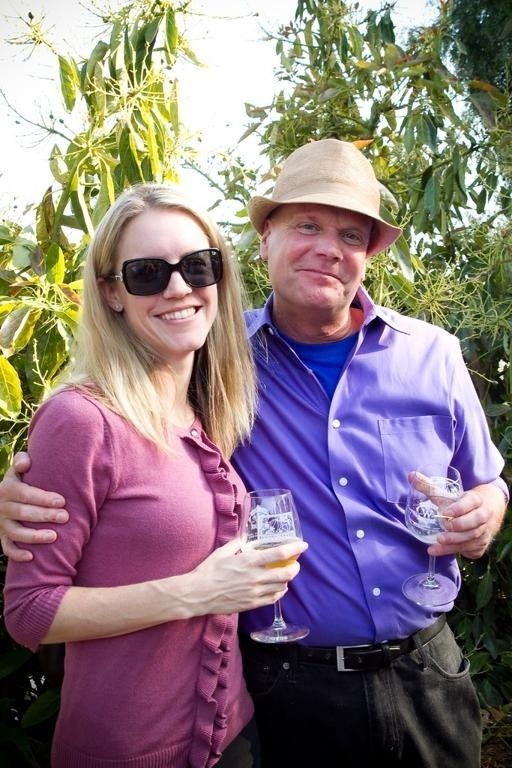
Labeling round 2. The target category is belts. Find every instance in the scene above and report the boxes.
[286,612,447,672]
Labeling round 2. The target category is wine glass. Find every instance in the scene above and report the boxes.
[402,462,464,607]
[239,487,310,643]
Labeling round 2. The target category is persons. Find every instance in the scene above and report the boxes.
[1,181,309,767]
[0,139,510,768]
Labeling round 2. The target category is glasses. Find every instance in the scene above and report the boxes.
[107,247,224,295]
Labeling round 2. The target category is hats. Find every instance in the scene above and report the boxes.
[248,138,402,260]
